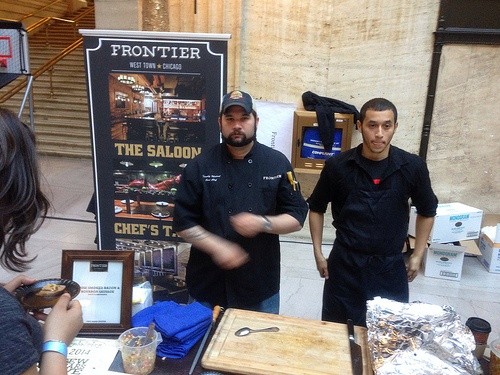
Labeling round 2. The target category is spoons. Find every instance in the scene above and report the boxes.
[235,327,279,337]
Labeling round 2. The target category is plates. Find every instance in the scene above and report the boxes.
[156,201,168,206]
[121,200,134,203]
[16,279,81,309]
[151,211,170,217]
[114,206,122,214]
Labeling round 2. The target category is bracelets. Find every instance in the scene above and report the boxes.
[39,339,68,357]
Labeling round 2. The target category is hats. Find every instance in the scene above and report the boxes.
[222,89,253,114]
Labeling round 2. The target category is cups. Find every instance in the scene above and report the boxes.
[117,327,162,375]
[465,317,491,361]
[489,339,500,375]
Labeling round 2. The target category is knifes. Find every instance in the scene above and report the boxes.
[347,319,363,375]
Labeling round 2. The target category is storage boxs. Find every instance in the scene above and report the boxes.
[409,235,483,283]
[478,226,500,274]
[409,201,484,244]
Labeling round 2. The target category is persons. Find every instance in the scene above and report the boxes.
[305,98,439,328]
[0,108,84,375]
[172,90,309,315]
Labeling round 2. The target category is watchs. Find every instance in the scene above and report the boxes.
[260,214,272,233]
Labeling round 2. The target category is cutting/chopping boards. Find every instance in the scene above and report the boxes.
[201,308,373,375]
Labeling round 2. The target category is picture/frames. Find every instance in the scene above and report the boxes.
[59,250,134,334]
